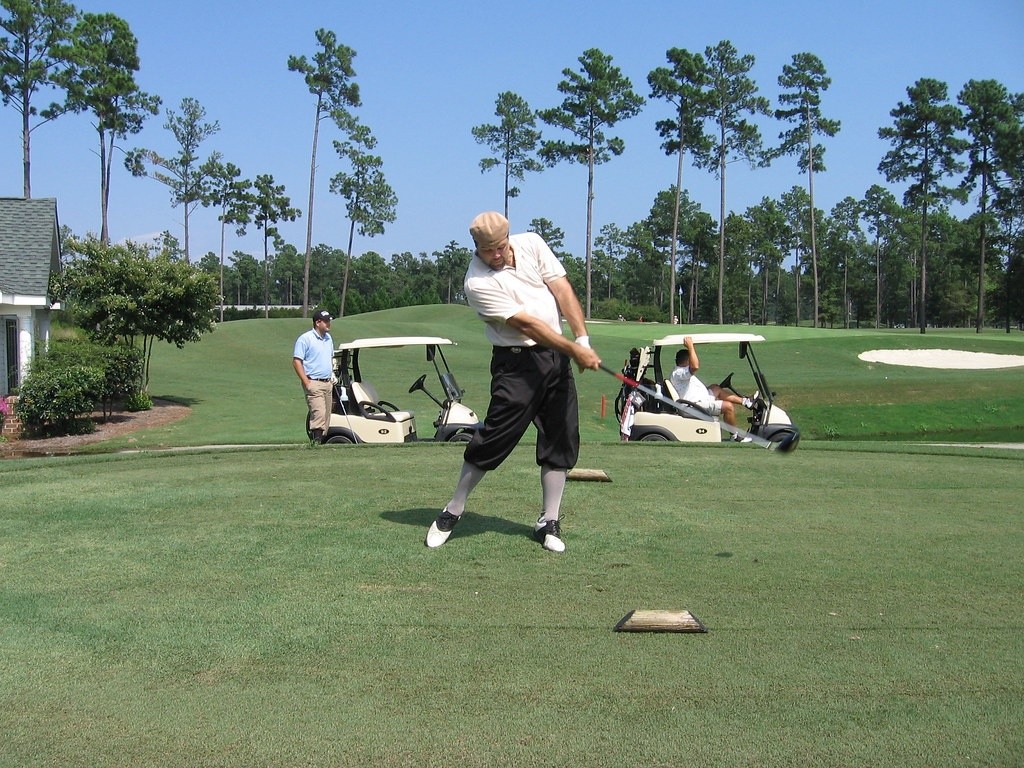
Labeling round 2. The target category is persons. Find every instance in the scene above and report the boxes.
[426,211,602,552]
[670,337,760,442]
[292,310,338,446]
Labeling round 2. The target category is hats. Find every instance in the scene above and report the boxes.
[470,211,509,246]
[313,310,334,322]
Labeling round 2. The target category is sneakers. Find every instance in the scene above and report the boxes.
[533,514,566,552]
[426,501,464,547]
[730,432,752,443]
[745,391,760,410]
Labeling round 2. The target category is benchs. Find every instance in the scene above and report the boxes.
[353,380,415,422]
[664,379,721,421]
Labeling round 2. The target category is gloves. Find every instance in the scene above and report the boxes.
[331,373,338,385]
[308,383,320,396]
[574,336,592,374]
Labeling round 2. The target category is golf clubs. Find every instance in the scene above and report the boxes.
[594,360,799,459]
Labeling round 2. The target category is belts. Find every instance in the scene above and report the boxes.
[308,377,331,382]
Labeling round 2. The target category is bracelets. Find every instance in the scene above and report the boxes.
[575,336,590,349]
